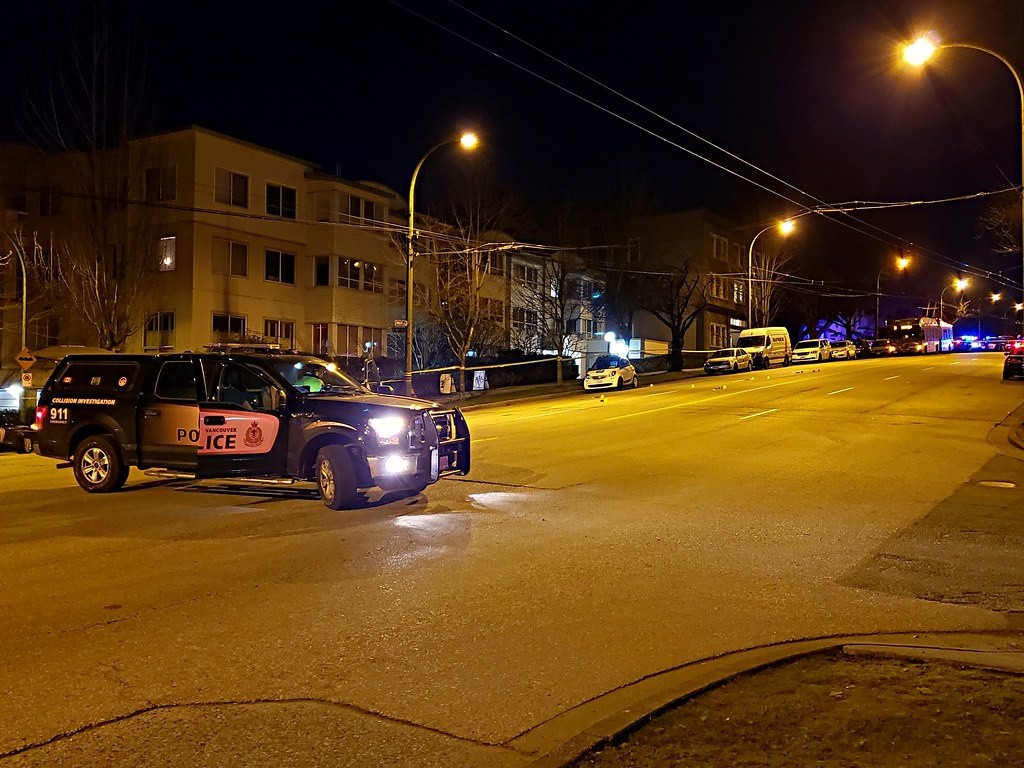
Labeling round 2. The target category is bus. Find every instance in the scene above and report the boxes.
[893,318,954,353]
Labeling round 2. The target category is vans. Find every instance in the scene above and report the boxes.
[736,326,792,369]
[792,339,832,363]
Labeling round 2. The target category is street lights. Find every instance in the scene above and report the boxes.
[394,127,478,396]
[941,281,966,319]
[1001,305,1021,336]
[749,222,793,328]
[604,332,615,353]
[979,295,998,340]
[896,32,1024,298]
[876,258,906,339]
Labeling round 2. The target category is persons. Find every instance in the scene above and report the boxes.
[292,362,327,393]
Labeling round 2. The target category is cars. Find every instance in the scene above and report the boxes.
[1002,353,1024,381]
[583,356,639,391]
[703,347,753,373]
[956,336,1024,351]
[830,341,857,360]
[857,339,922,356]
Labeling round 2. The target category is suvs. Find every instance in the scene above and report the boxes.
[32,352,471,511]
